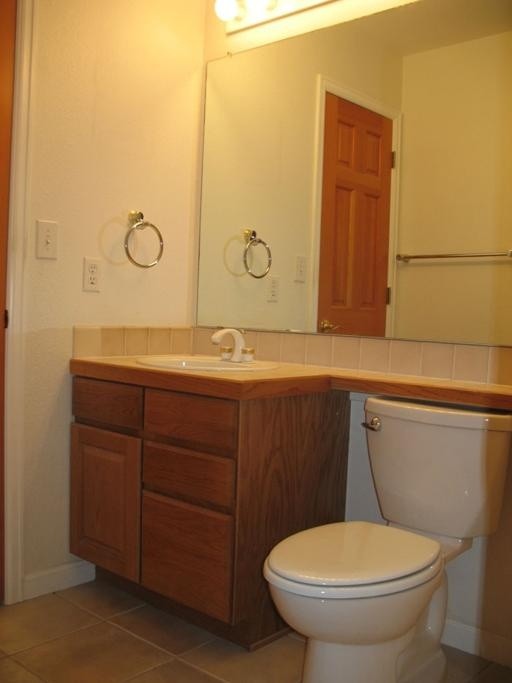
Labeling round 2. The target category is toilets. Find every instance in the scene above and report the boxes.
[262,394,512,683]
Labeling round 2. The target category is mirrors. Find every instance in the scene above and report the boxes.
[195,0,512,348]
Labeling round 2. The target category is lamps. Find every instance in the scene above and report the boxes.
[215,0,332,35]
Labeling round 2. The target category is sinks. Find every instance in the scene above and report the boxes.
[137,359,279,370]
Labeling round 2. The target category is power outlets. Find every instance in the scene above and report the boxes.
[83,256,102,292]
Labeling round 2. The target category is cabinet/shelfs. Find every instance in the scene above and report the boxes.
[70,378,349,653]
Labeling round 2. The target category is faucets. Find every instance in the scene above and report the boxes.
[211,328,246,362]
